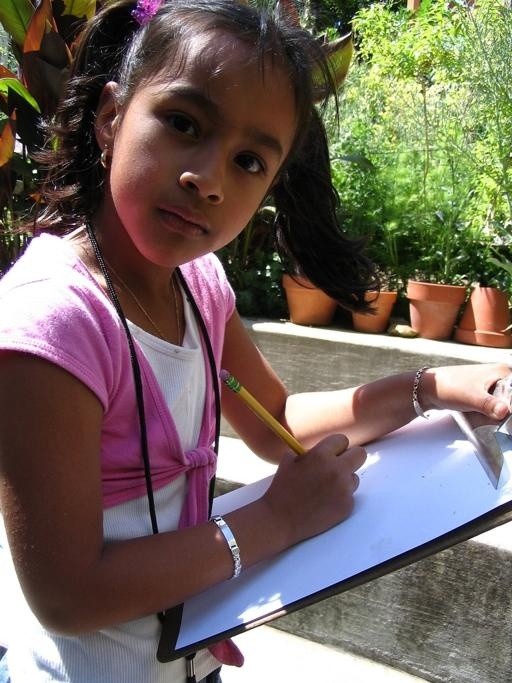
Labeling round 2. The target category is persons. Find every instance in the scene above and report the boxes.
[0,1,511,682]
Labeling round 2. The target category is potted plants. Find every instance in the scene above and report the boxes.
[274,203,511,349]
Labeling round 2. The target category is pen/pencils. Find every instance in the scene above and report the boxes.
[220,369,307,456]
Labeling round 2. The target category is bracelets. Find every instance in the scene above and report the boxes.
[207,514,243,582]
[412,366,432,421]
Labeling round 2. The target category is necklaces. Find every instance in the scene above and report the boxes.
[81,225,180,349]
